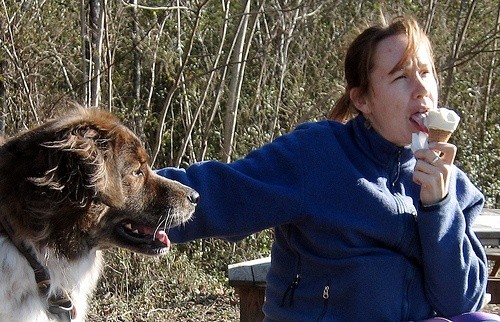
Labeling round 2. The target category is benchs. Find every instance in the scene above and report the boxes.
[226,222,500,322]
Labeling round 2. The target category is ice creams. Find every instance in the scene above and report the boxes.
[424,107,460,156]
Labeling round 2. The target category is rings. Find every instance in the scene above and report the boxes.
[430,156,440,165]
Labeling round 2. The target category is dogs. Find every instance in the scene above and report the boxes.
[0,105,200,322]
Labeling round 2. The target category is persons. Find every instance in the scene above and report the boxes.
[152,17,500,322]
